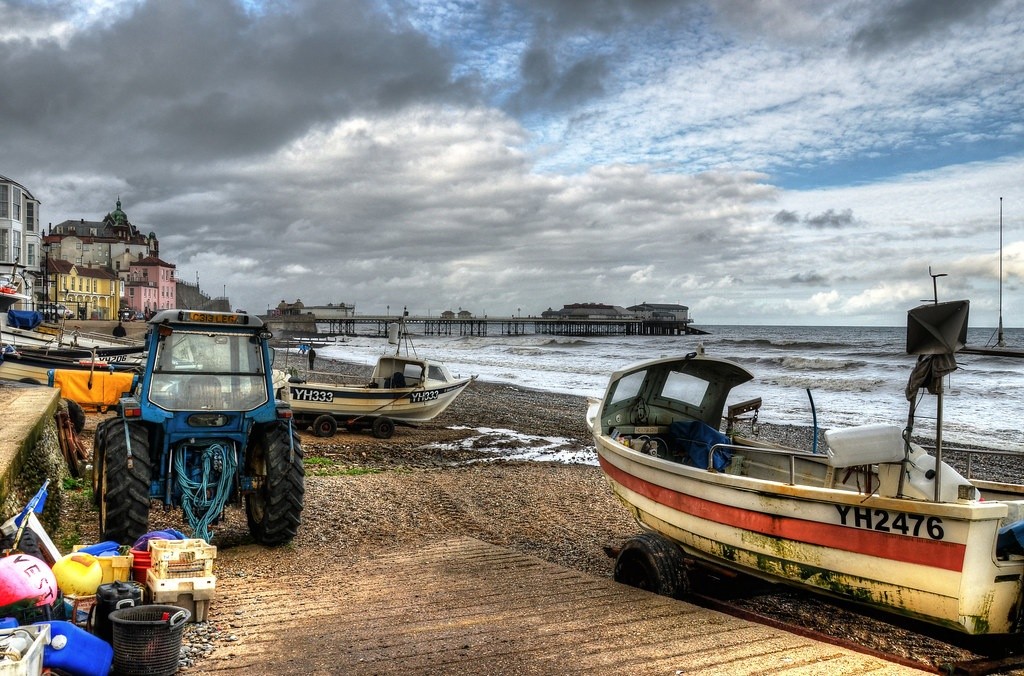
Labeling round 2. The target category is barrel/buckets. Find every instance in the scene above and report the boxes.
[94,580,144,645]
[132,548,151,584]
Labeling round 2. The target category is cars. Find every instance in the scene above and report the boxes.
[36,302,76,320]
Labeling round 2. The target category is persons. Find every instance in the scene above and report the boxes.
[113,322,126,337]
[309,346,316,370]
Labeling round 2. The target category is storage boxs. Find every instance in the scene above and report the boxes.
[73,539,217,631]
[0,592,65,676]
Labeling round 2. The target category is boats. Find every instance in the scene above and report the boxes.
[0,301,480,422]
[582,295,1024,646]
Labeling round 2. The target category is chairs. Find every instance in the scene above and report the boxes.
[185,376,223,410]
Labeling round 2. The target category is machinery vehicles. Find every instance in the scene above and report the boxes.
[90,311,305,547]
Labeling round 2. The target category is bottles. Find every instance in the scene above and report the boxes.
[824,425,906,465]
[32,621,113,676]
[908,456,981,503]
[0,618,18,628]
[878,442,927,500]
